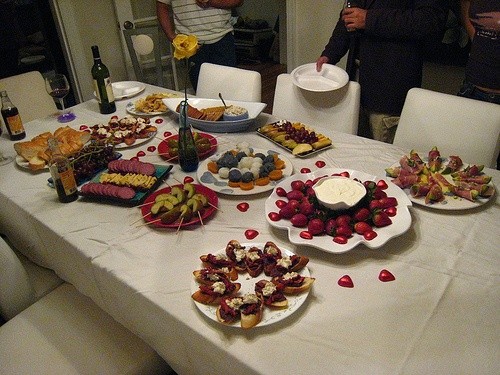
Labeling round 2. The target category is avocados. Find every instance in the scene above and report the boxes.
[168,130,211,155]
[151,183,209,224]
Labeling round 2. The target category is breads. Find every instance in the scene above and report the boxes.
[191,239,315,329]
[91,117,157,145]
[13,124,91,170]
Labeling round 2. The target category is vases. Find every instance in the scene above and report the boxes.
[178,101,199,172]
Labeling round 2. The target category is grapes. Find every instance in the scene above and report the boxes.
[65,144,116,182]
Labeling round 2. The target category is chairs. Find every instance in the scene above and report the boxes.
[196,62,261,103]
[0,237,65,321]
[271,74,360,136]
[393,88,500,169]
[0,281,169,375]
[0,71,57,130]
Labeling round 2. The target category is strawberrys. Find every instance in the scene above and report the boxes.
[279,172,398,235]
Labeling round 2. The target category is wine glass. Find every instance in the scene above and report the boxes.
[45,74,75,123]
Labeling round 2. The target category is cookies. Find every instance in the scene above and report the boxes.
[176,102,216,122]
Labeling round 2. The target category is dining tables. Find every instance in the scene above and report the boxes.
[0,83,500,374]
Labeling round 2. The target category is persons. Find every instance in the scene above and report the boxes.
[455,0,500,105]
[156,0,243,93]
[315,0,450,145]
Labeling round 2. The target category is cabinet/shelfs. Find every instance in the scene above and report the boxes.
[233,28,272,64]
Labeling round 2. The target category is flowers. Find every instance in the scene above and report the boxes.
[172,34,199,163]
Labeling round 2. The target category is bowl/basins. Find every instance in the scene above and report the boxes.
[161,97,267,133]
[312,175,367,210]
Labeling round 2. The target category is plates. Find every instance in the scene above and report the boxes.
[126,95,167,116]
[191,243,311,328]
[105,125,156,148]
[47,151,122,187]
[197,147,293,195]
[255,119,334,158]
[157,131,217,161]
[139,184,220,227]
[265,168,413,255]
[95,80,148,100]
[15,153,51,170]
[76,158,173,205]
[291,62,349,92]
[386,157,496,210]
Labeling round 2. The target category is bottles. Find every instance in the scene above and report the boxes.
[90,45,116,114]
[46,136,81,203]
[0,90,26,141]
